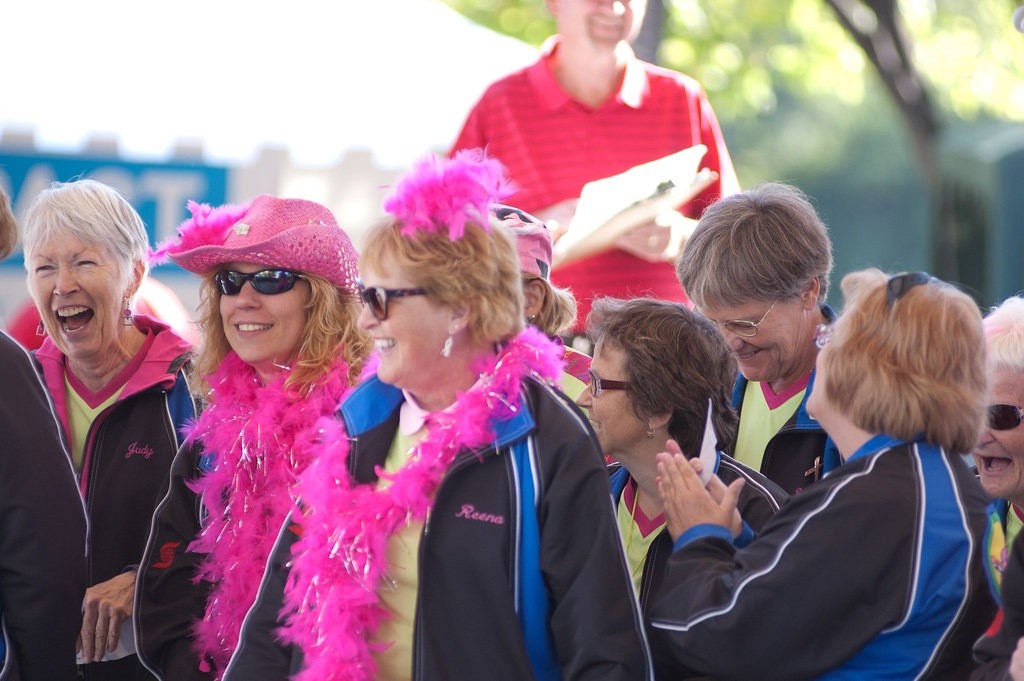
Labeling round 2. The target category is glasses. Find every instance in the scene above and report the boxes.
[987,403,1024,430]
[886,271,930,308]
[214,268,302,295]
[587,369,631,399]
[706,300,779,337]
[816,324,832,348]
[356,284,424,323]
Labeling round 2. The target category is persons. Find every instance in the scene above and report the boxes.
[134,195,377,681]
[489,203,593,404]
[450,0,741,327]
[224,147,650,681]
[972,297,1023,681]
[643,269,1007,681]
[0,329,90,681]
[574,295,789,622]
[20,177,215,681]
[677,182,842,496]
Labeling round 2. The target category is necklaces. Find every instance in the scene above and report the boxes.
[624,486,668,578]
[176,347,355,680]
[270,326,566,681]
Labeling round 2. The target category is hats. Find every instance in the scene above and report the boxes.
[164,195,361,298]
[487,203,555,281]
